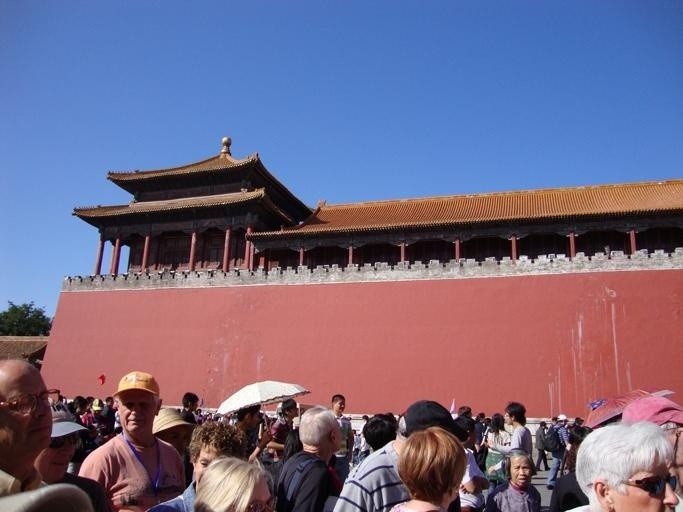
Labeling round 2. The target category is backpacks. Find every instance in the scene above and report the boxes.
[543,426,561,452]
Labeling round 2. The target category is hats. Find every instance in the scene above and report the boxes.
[152,408,191,435]
[47,397,88,438]
[398,400,467,442]
[557,415,570,422]
[112,371,159,398]
[622,395,683,426]
[92,399,104,411]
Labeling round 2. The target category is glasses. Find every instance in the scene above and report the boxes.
[622,476,676,495]
[50,435,81,448]
[249,496,278,512]
[0,389,59,414]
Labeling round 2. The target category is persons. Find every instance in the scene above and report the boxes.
[0,359,488,512]
[456,394,682,512]
